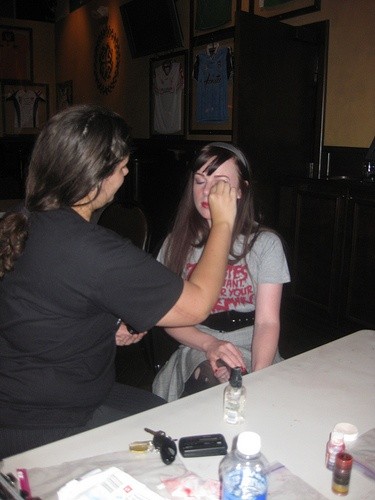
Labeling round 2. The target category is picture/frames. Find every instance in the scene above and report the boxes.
[0,0,322,144]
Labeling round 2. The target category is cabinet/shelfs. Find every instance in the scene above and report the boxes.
[293,170,375,336]
[0,133,39,200]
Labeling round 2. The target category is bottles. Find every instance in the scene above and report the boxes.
[220,431,268,500]
[331,452,353,495]
[223,366,246,425]
[325,431,346,469]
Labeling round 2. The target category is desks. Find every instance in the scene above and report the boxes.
[0,330,375,500]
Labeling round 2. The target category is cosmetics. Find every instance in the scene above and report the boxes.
[224,368,246,423]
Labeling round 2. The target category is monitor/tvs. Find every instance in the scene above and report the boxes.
[119,0,185,61]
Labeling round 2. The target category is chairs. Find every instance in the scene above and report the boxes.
[99,200,159,372]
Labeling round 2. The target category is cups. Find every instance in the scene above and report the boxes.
[331,423,359,446]
[360,159,375,184]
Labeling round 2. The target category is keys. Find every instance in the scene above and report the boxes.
[143,426,177,465]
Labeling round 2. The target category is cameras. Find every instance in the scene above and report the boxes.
[178,433,229,459]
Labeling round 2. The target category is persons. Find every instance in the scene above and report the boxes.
[152,143,291,399]
[0,104,237,455]
